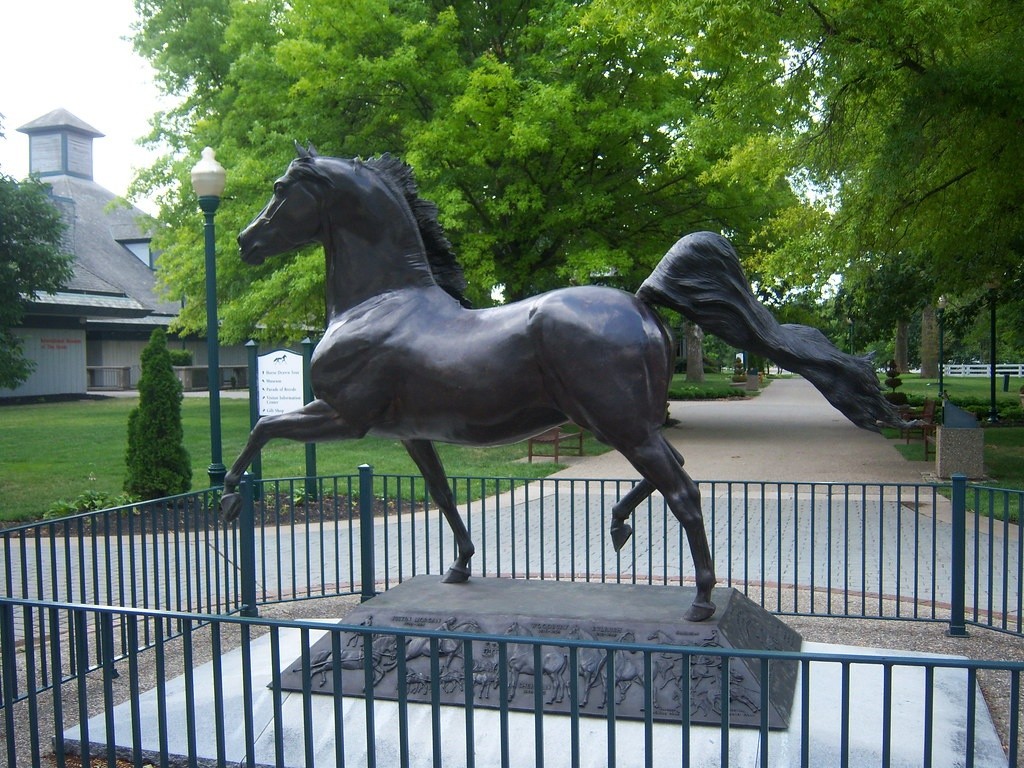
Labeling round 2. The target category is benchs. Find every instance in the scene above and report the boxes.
[922,423,936,461]
[898,397,936,446]
[527,425,586,464]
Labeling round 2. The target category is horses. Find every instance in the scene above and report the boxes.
[310,618,762,717]
[216,140,930,622]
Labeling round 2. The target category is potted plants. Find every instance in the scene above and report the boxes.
[729,357,747,391]
[1019,384,1024,404]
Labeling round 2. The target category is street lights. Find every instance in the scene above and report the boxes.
[189,145,228,510]
[847,317,854,354]
[937,296,947,398]
[983,271,1001,422]
